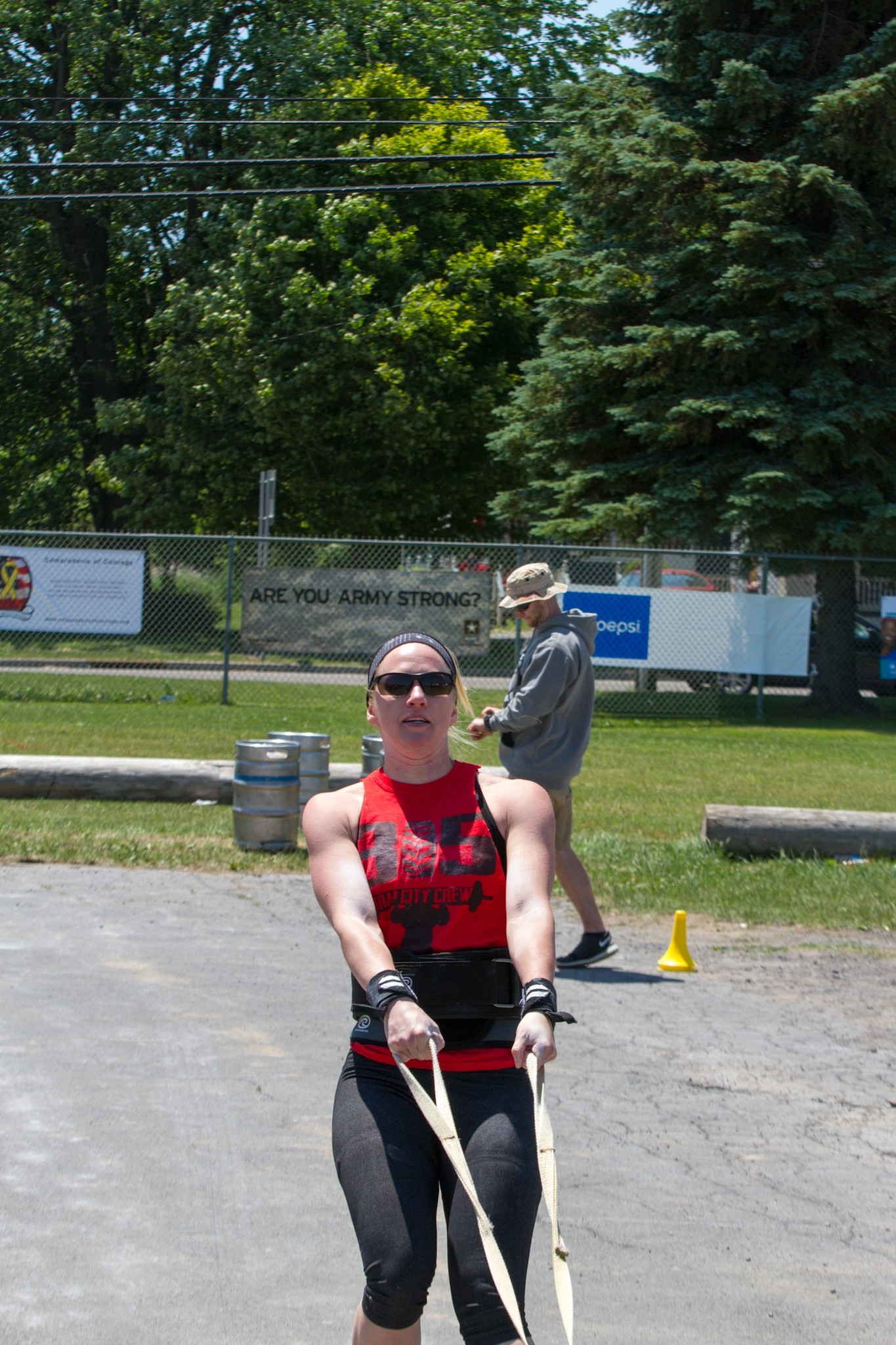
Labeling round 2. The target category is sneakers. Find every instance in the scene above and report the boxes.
[556,930,619,967]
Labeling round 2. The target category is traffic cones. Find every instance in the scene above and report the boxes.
[657,909,698,974]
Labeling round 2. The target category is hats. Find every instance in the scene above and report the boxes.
[498,561,569,608]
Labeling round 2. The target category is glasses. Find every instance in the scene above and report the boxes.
[513,601,532,613]
[367,671,456,698]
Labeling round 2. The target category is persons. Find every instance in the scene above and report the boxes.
[451,550,507,626]
[150,557,204,577]
[303,632,557,1345]
[879,612,896,679]
[467,563,619,966]
[735,555,778,594]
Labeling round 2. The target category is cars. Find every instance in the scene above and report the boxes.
[685,596,882,695]
[617,569,715,591]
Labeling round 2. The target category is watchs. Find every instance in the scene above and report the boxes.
[484,714,492,731]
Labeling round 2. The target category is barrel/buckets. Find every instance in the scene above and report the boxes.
[231,740,301,852]
[359,733,386,780]
[267,732,331,807]
